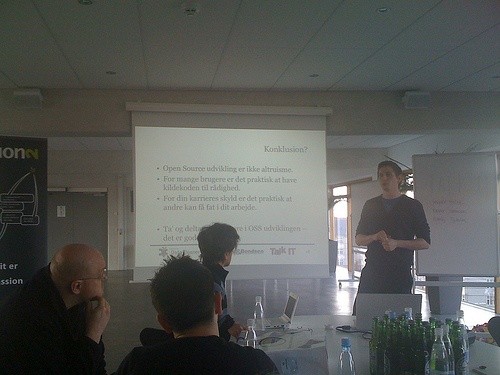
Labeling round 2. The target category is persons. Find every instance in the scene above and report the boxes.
[352,160,432,317]
[0,242,109,375]
[114,222,280,375]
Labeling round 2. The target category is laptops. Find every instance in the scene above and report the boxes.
[262,292,299,328]
[355,293,422,331]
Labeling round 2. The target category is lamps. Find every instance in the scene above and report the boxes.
[401,90,436,110]
[11,87,43,109]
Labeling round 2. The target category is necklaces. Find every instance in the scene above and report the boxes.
[175,332,193,339]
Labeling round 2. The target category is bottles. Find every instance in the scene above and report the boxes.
[245,318,256,347]
[369,306,470,375]
[337,338,355,374]
[253,295,263,329]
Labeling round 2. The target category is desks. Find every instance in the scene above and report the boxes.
[228,315,500,375]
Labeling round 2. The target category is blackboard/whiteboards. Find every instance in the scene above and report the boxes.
[350,179,383,248]
[411,151,500,277]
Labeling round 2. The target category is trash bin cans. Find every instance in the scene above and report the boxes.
[426,277,464,317]
[329,240,338,275]
